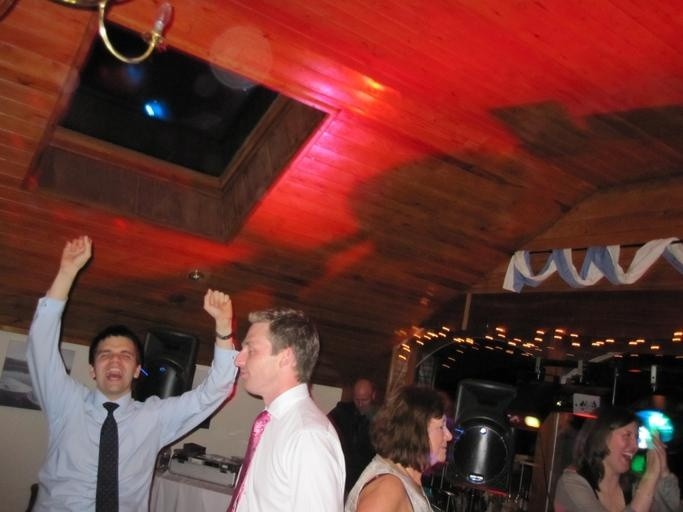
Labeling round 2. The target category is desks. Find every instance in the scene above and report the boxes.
[149,467,234,512]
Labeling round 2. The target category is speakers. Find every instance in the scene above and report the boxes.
[131,329,199,403]
[447,377,523,495]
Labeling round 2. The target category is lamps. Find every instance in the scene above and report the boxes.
[497,379,567,512]
[57,0,174,66]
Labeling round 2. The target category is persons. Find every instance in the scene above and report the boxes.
[223,306,348,511]
[551,404,681,512]
[342,383,454,512]
[324,375,383,506]
[21,234,240,511]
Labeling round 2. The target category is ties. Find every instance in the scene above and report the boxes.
[95,401,119,512]
[225,409,272,512]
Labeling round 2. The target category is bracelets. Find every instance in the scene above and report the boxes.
[213,330,233,340]
